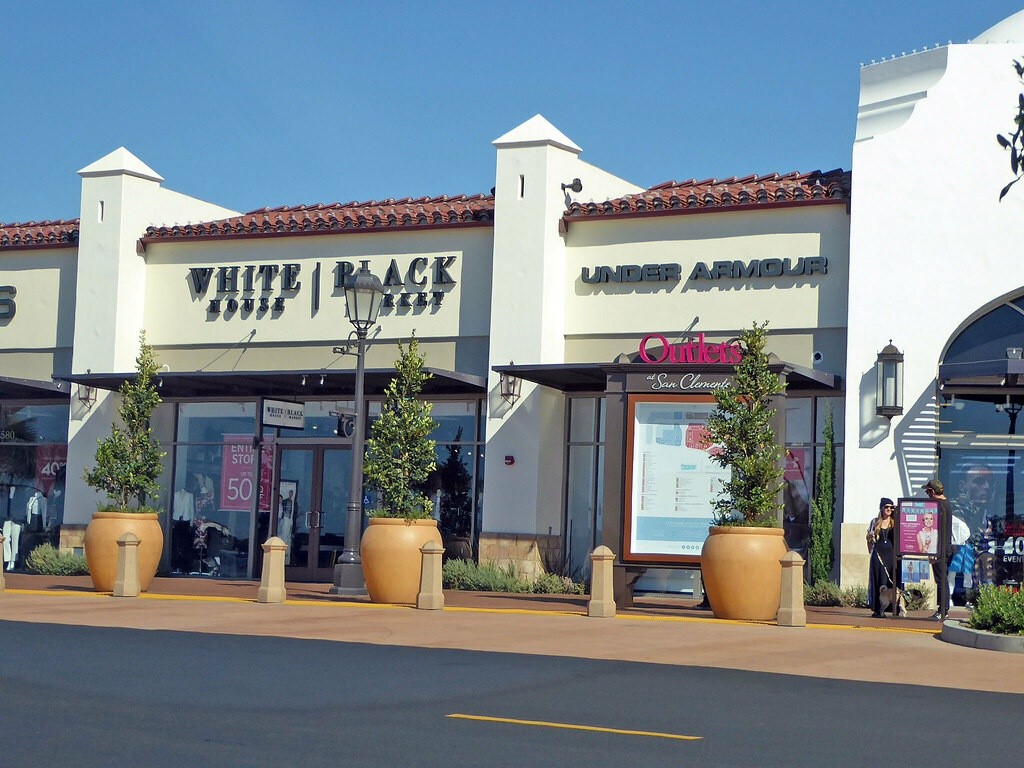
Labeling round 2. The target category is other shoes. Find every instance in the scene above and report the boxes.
[933,611,949,619]
[872,611,886,618]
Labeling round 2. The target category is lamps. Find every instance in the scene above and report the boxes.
[875,339,904,419]
[501,359,524,408]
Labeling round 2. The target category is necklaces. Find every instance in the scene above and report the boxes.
[881,523,889,543]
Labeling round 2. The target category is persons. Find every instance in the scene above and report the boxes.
[948,460,997,593]
[867,498,904,618]
[26,491,47,572]
[193,487,213,576]
[947,517,976,606]
[172,488,194,574]
[282,490,293,559]
[277,495,283,537]
[917,512,938,553]
[45,489,63,548]
[1,486,25,571]
[921,479,954,620]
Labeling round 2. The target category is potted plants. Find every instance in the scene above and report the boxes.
[359,329,443,605]
[85,330,169,594]
[698,319,794,623]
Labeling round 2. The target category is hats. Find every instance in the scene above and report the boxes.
[921,479,943,494]
[879,497,893,508]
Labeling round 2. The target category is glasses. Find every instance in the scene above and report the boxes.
[885,506,894,509]
[925,490,931,494]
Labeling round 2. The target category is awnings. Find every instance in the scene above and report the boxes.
[0,376,69,398]
[53,368,487,395]
[492,342,842,392]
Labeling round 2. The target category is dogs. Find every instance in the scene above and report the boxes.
[879,585,922,618]
